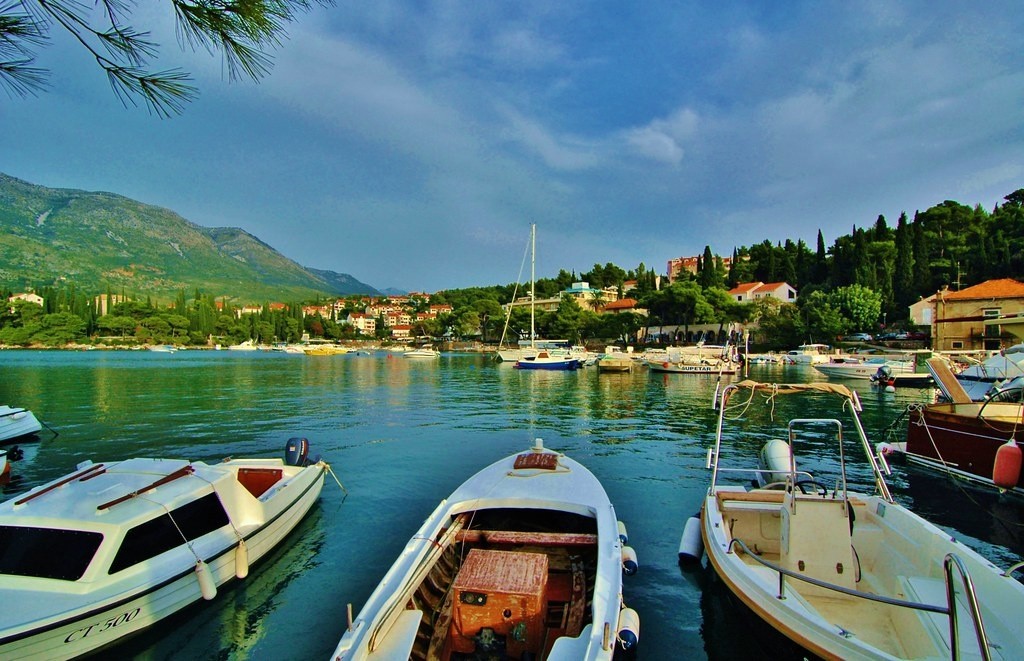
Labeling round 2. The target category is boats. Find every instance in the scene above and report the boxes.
[0,438,345,661]
[678,380,1024,661]
[0,405,42,486]
[403,344,440,358]
[228,341,348,356]
[329,438,640,661]
[146,345,177,352]
[491,224,1024,557]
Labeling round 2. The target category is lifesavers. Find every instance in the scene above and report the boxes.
[723,358,730,363]
[677,363,684,369]
